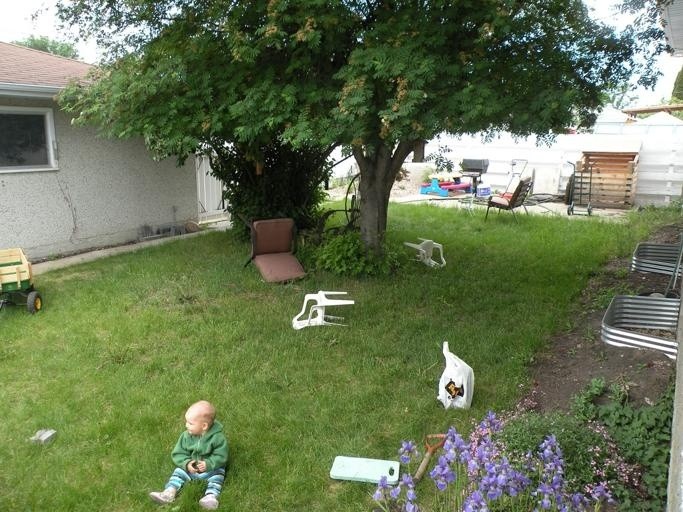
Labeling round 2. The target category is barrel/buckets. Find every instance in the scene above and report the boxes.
[477,184,492,197]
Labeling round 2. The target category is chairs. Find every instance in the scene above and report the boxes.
[292,289,357,332]
[401,234,447,271]
[484,175,534,225]
[233,208,307,285]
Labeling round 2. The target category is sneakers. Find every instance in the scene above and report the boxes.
[198,493,220,510]
[149,491,175,506]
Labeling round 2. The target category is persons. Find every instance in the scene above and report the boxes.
[148,399,231,510]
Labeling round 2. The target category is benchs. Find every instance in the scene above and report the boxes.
[440,183,473,197]
[419,181,451,194]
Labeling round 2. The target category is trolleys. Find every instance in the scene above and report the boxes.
[565,159,599,216]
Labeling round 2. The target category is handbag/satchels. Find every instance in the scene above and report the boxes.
[437,341,475,410]
